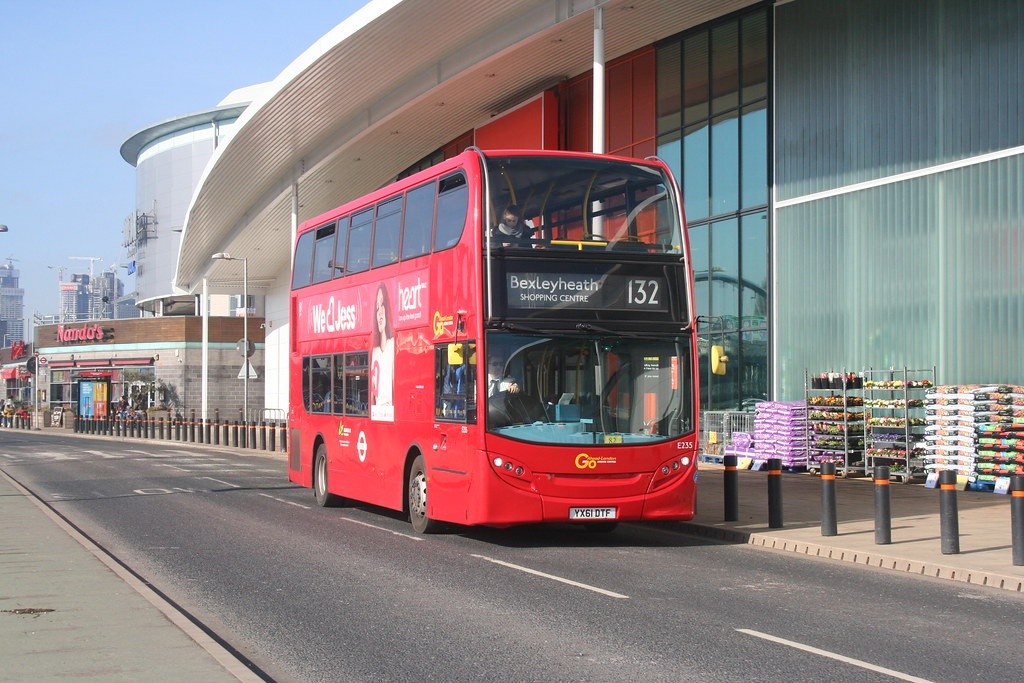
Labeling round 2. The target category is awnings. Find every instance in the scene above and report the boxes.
[0,368,15,379]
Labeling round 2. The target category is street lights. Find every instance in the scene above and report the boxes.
[3,333,11,349]
[211,253,250,427]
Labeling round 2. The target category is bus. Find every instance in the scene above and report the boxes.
[285,145,728,535]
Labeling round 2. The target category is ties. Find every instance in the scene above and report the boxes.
[490,379,499,396]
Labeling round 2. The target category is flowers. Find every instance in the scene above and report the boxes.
[810,372,932,472]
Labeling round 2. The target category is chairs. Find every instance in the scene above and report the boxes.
[582,234,646,251]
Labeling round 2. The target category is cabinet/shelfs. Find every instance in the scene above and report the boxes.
[861,364,937,485]
[804,367,873,479]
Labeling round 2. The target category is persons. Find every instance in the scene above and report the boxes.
[0,395,15,428]
[370,282,394,406]
[163,397,176,418]
[474,352,520,405]
[121,394,148,411]
[492,205,535,249]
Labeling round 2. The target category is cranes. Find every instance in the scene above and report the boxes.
[67,255,105,286]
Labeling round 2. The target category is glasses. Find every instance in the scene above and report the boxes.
[489,362,504,367]
